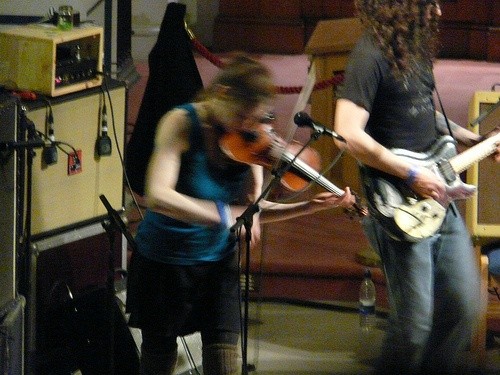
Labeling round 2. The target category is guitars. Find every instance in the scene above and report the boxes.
[358,133,500,243]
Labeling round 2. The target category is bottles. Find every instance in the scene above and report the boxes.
[358,271,377,331]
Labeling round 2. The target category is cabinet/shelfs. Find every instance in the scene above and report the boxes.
[305,18,358,197]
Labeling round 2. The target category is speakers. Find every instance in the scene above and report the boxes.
[461,90,500,249]
[14,76,129,242]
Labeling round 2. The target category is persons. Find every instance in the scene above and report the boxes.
[331,0,500,375]
[126,53,356,375]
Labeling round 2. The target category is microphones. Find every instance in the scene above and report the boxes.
[45,113,58,164]
[293,112,346,142]
[97,104,113,154]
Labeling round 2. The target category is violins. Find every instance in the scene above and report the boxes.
[218,106,369,221]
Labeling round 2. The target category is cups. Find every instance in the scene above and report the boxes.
[57,5,73,32]
